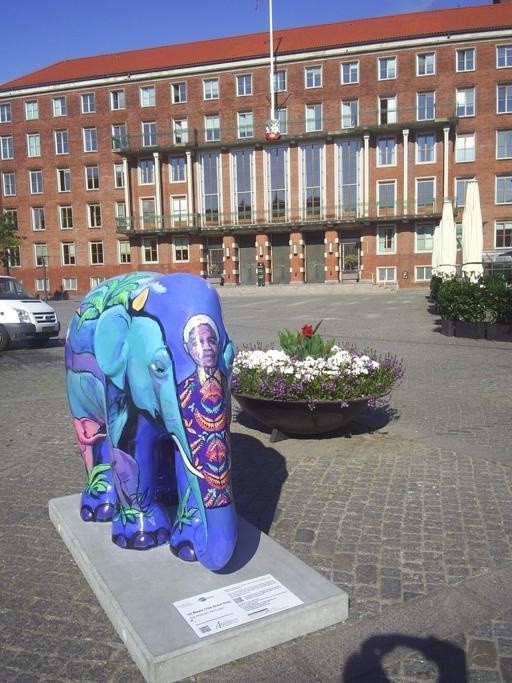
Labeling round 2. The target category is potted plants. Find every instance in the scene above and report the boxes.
[429,269,512,341]
[205,263,223,286]
[341,254,359,284]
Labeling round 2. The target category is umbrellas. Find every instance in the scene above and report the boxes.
[431,176,483,289]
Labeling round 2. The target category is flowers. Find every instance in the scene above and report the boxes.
[230,318,407,413]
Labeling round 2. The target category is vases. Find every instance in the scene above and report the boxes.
[231,387,393,443]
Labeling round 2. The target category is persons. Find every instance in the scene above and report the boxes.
[176,311,234,509]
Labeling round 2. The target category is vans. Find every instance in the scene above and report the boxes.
[0,275,61,350]
